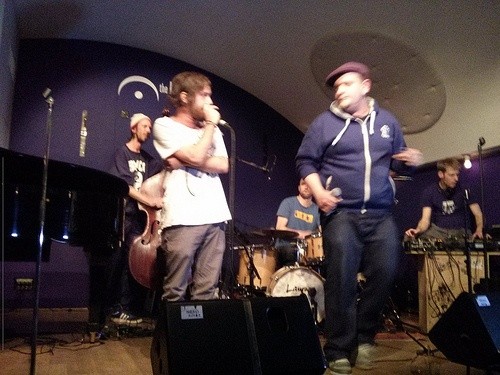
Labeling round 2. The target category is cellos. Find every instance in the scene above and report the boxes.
[128,169,166,290]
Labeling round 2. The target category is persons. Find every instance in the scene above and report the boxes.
[404,157,492,243]
[89,113,162,325]
[295,62,423,375]
[276,177,322,240]
[152,72,228,302]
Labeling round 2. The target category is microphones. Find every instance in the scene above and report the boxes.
[330,188,343,197]
[219,119,231,129]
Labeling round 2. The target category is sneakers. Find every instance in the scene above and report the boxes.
[355,343,373,370]
[111,311,143,323]
[328,358,352,375]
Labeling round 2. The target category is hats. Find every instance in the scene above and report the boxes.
[130,113,152,129]
[325,61,371,89]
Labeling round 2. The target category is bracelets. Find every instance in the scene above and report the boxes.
[205,120,218,128]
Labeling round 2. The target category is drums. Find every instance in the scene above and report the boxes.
[303,232,324,265]
[237,249,276,288]
[267,265,326,325]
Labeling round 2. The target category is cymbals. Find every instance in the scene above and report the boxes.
[262,230,299,238]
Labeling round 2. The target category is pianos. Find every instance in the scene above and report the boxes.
[403,237,500,336]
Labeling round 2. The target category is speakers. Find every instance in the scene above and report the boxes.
[428,291,500,372]
[150,293,329,375]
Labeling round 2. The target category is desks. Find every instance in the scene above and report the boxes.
[404,251,500,333]
